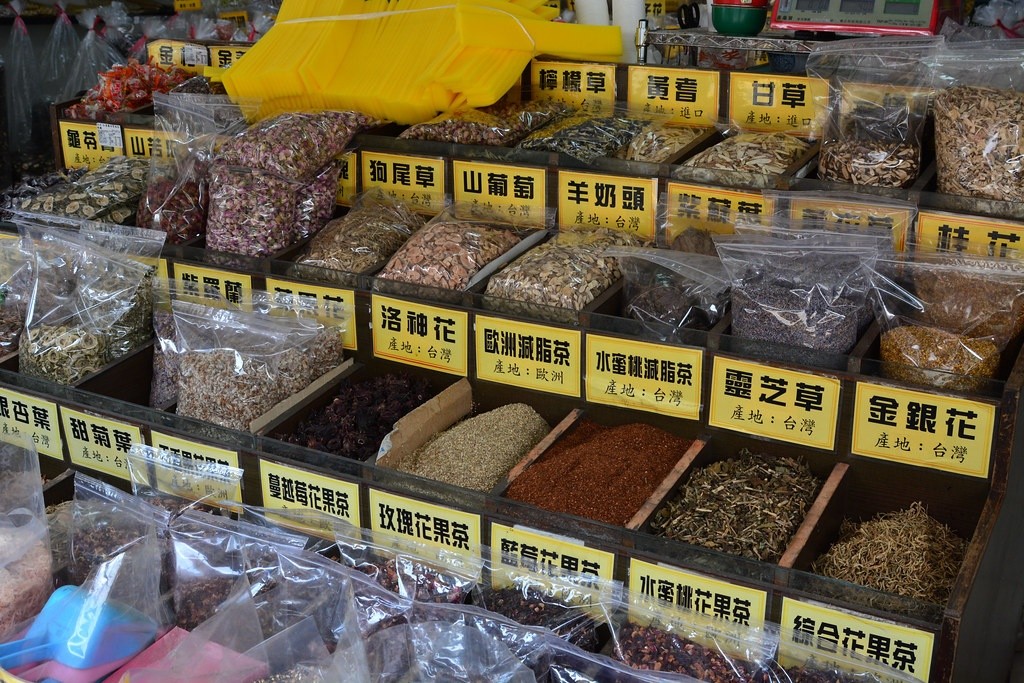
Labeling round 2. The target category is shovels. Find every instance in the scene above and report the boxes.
[0,585,159,669]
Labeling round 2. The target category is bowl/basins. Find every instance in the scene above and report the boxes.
[711,0,768,36]
